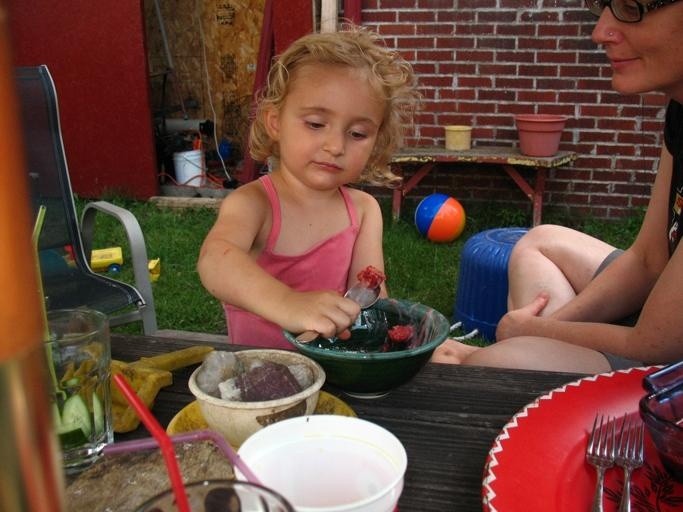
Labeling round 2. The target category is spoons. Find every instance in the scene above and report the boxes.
[293,283,383,344]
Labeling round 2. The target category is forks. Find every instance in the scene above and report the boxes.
[613,412,648,511]
[479,361,683,512]
[584,412,618,512]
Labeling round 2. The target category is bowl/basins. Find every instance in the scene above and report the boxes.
[282,296,451,401]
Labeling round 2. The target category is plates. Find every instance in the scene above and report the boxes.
[163,392,366,447]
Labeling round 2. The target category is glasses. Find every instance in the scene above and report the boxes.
[581,1,683,25]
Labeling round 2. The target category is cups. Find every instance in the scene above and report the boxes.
[43,307,113,480]
[229,415,408,511]
[187,347,327,449]
[133,478,298,512]
[641,360,683,485]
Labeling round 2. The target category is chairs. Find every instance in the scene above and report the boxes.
[15,64,157,338]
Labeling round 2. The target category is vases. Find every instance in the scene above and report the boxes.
[513,114,568,158]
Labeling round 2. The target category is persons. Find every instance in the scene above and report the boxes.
[462,1,681,370]
[197,16,479,365]
